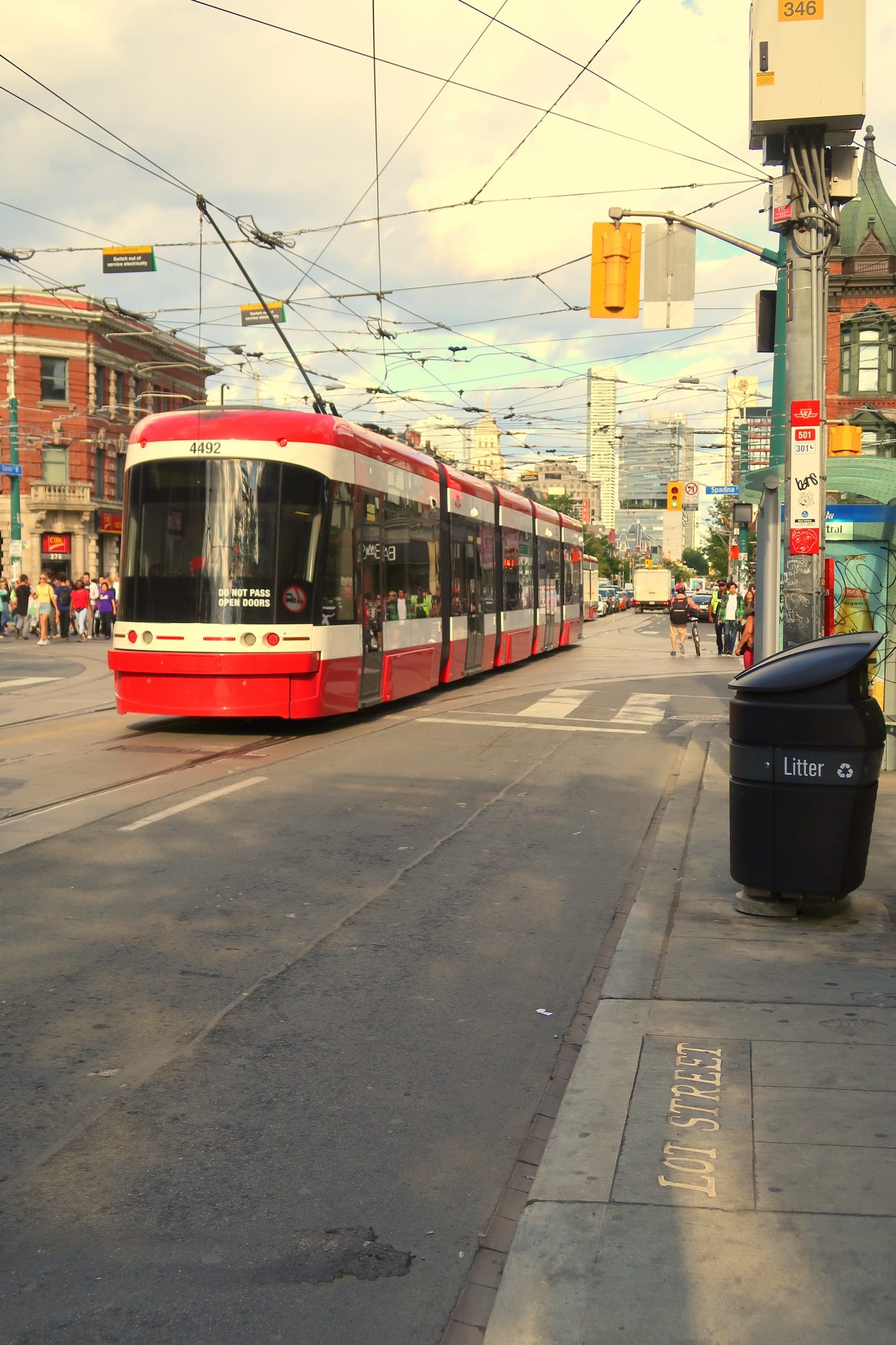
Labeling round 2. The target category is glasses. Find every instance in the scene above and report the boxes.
[718,584,725,587]
[40,577,45,580]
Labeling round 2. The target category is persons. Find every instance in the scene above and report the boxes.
[30,574,59,646]
[708,580,728,654]
[669,584,704,656]
[0,571,120,642]
[717,582,744,657]
[735,611,755,669]
[744,584,755,607]
[358,586,461,652]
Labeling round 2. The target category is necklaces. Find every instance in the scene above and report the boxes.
[747,601,751,609]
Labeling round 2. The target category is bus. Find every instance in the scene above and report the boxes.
[583,554,599,621]
[106,403,585,721]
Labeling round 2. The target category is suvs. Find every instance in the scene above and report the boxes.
[598,588,614,615]
[601,588,620,613]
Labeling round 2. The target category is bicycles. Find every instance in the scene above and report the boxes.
[686,613,701,656]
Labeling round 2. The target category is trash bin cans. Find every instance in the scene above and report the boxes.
[727,631,887,918]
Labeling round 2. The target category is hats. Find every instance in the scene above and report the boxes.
[717,580,727,585]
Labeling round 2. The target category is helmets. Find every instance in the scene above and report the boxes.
[676,583,684,592]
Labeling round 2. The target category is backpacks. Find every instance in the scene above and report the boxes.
[670,596,690,624]
[58,585,71,605]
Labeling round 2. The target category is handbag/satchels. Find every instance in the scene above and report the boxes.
[94,610,101,619]
[27,596,40,617]
[69,607,76,620]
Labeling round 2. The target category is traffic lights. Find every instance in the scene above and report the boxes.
[733,502,752,522]
[666,482,683,511]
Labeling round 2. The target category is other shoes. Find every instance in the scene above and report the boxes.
[47,634,52,638]
[104,635,109,640]
[678,643,685,654]
[32,627,39,637]
[14,628,19,640]
[53,633,60,638]
[22,637,29,640]
[71,629,78,635]
[718,651,723,654]
[77,636,87,643]
[721,651,727,656]
[36,638,49,646]
[61,638,69,640]
[95,634,99,637]
[670,651,676,656]
[727,651,733,657]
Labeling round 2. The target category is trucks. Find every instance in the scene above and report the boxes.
[633,569,673,614]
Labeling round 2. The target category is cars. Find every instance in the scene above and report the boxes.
[602,585,635,612]
[673,577,719,621]
[598,594,608,618]
